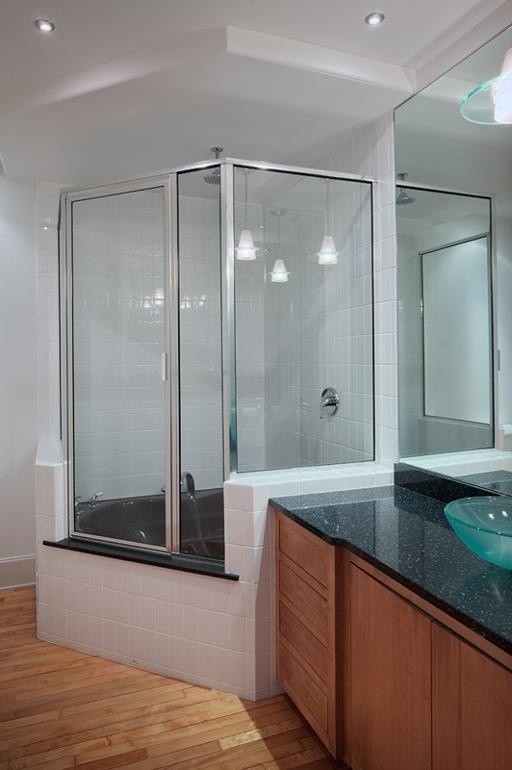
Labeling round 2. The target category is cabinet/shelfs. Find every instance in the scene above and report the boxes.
[340,548,508,770]
[270,508,341,766]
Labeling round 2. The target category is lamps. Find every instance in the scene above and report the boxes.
[460,47,512,126]
[234,167,341,283]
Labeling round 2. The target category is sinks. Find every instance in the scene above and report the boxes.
[445,496,512,573]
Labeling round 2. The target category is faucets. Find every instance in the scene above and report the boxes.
[163,474,196,499]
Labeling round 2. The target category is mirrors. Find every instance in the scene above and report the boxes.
[417,232,491,428]
[392,22,512,497]
